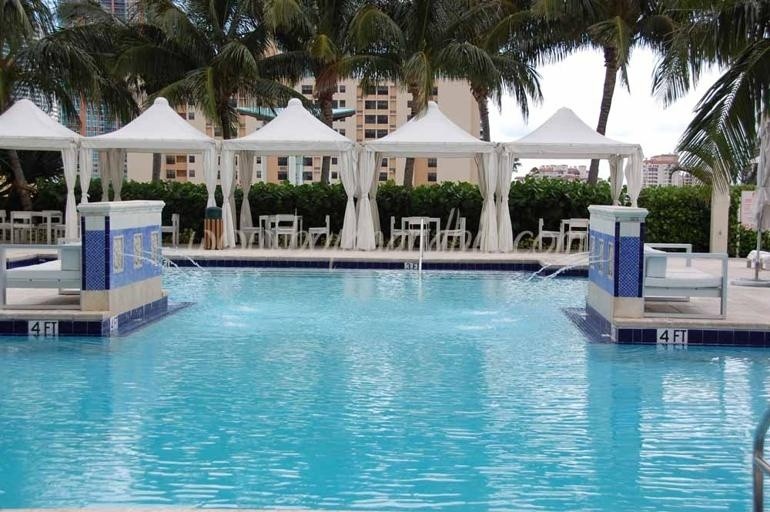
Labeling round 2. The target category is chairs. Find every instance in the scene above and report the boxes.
[643,243,728,319]
[539,218,589,253]
[162,213,179,248]
[0,210,65,245]
[391,216,466,251]
[243,214,331,249]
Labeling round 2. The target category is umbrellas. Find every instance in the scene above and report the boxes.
[744,116,770,279]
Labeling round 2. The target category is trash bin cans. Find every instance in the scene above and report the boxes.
[204,207,224,249]
